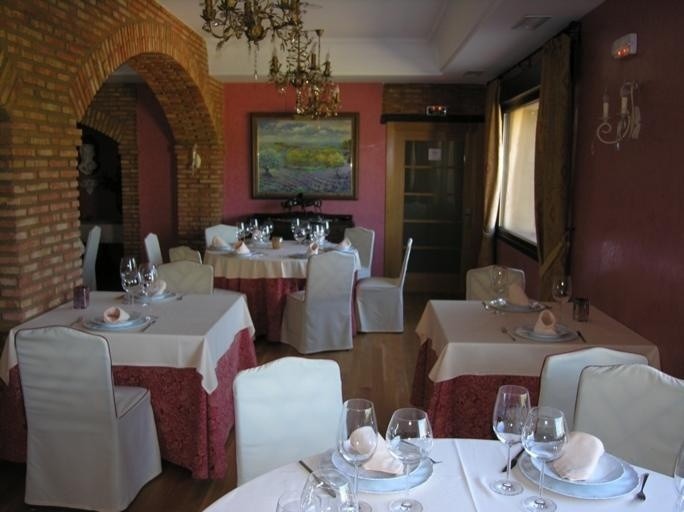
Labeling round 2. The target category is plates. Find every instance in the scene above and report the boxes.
[74,285,177,333]
[206,244,234,252]
[531,441,626,487]
[517,449,639,501]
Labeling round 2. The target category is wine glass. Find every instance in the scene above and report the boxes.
[118,255,157,313]
[485,264,591,343]
[277,383,568,512]
[237,217,332,259]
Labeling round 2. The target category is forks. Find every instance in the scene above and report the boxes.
[634,472,652,501]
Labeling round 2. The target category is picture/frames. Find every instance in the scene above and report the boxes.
[249,111,360,200]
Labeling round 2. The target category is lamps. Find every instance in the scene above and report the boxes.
[587,80,656,155]
[200,0,342,121]
[191,145,201,175]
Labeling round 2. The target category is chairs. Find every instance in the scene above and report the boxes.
[83,226,102,291]
[467,265,526,301]
[15,326,162,512]
[145,224,413,355]
[233,357,347,487]
[573,364,684,477]
[537,348,648,433]
